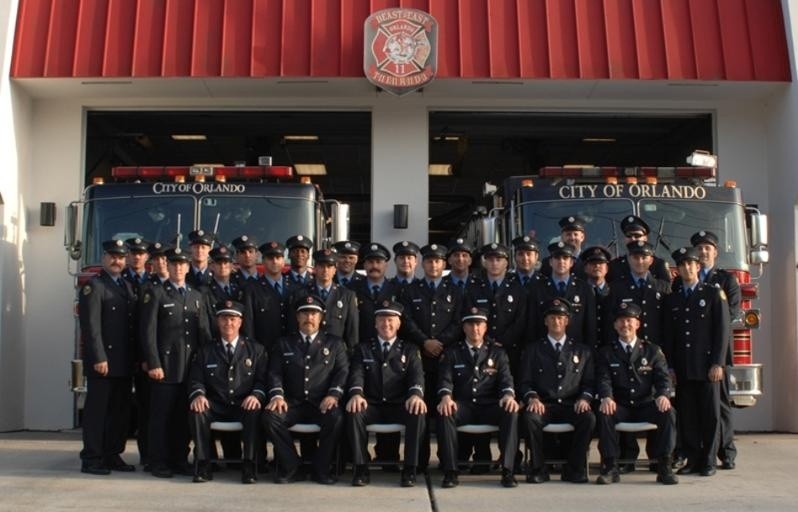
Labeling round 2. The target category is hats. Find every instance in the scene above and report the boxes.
[512,236,540,250]
[447,239,476,257]
[335,241,359,253]
[461,306,489,324]
[313,250,337,262]
[147,242,168,254]
[287,235,312,250]
[102,240,130,255]
[295,294,326,313]
[258,241,286,256]
[541,296,572,318]
[615,302,642,318]
[628,240,653,256]
[209,246,233,261]
[393,241,419,255]
[214,299,246,318]
[548,242,575,256]
[165,249,191,261]
[691,231,718,247]
[232,235,258,248]
[127,238,150,251]
[621,215,649,234]
[360,243,391,261]
[672,247,699,264]
[482,243,510,259]
[581,247,611,264]
[420,244,448,257]
[372,297,404,317]
[559,217,588,232]
[188,231,213,245]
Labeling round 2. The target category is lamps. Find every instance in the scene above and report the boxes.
[39,201,56,226]
[393,205,407,229]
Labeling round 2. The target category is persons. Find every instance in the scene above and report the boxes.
[79,214,741,488]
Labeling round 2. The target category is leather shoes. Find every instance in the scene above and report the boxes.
[79,449,741,488]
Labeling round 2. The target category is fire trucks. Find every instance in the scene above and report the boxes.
[59,154,353,444]
[453,148,770,412]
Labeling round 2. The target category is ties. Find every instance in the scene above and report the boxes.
[305,335,311,347]
[275,283,282,297]
[372,285,379,295]
[342,278,347,285]
[471,347,478,360]
[226,342,233,362]
[224,286,229,297]
[197,271,203,285]
[559,282,566,293]
[595,287,602,298]
[523,277,529,288]
[430,282,435,295]
[117,279,124,288]
[554,341,562,353]
[458,281,464,297]
[134,275,140,287]
[687,288,693,298]
[381,341,390,358]
[247,277,255,283]
[402,280,408,296]
[493,282,499,298]
[624,343,633,356]
[297,275,301,285]
[179,288,185,297]
[322,289,327,298]
[639,279,645,288]
